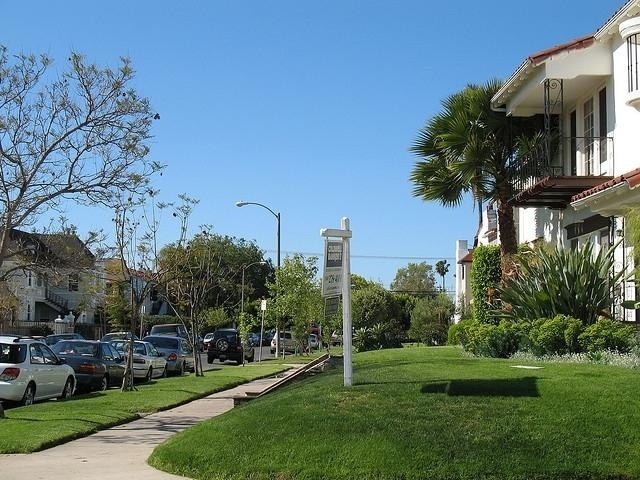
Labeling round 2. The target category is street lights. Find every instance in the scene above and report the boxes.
[237,201,280,360]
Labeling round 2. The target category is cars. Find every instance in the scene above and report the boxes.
[1,335,78,407]
[249,330,343,353]
[47,324,214,394]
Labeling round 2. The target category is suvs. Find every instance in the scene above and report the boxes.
[207,329,254,364]
